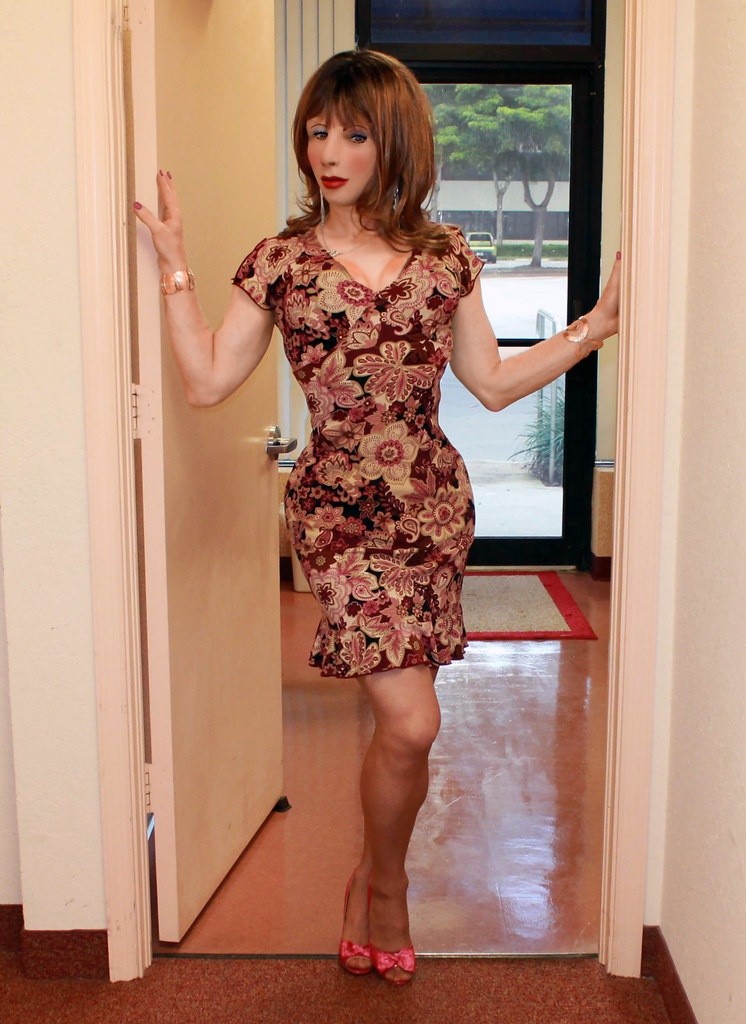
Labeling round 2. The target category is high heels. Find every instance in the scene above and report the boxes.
[337,866,416,986]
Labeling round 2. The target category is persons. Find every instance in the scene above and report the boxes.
[129,48,622,984]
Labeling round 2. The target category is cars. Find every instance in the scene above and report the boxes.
[464,232,499,263]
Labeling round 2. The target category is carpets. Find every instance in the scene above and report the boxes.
[460,571,599,641]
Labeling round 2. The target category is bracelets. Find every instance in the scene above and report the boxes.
[562,315,604,360]
[158,267,198,295]
